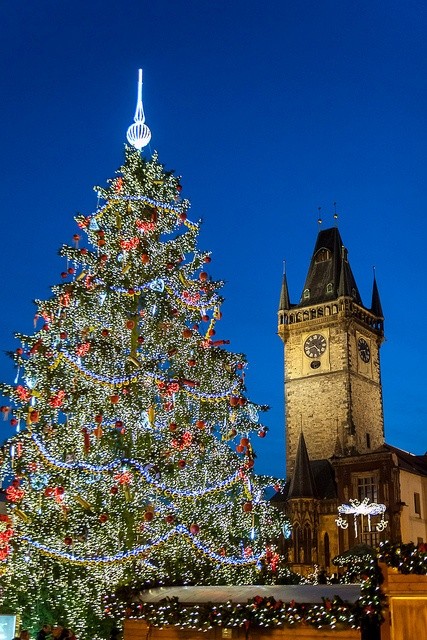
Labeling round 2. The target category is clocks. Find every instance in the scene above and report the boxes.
[357,337,371,363]
[303,333,327,358]
